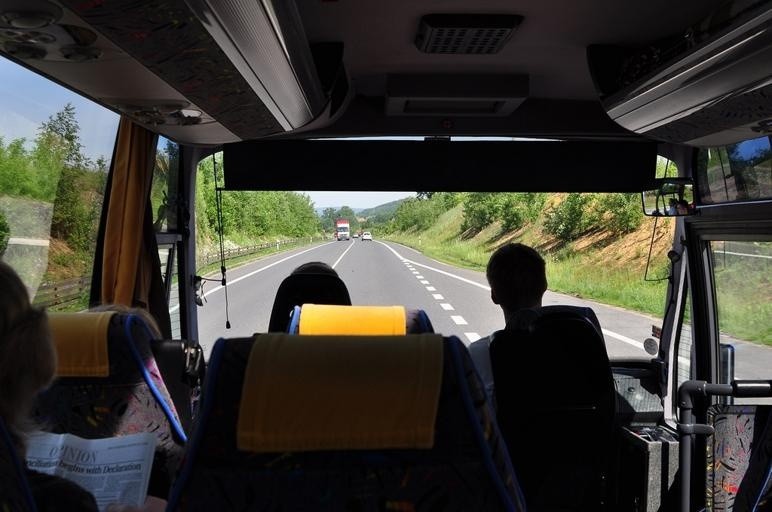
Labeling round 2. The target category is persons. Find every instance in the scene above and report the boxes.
[80,303,163,340]
[0,260,99,512]
[469,244,617,424]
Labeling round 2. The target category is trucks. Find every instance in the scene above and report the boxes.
[336,219,349,241]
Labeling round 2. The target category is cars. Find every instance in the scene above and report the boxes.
[362,231,372,241]
[353,234,358,238]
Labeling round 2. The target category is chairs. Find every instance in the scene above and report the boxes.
[189,305,523,512]
[34,309,206,479]
